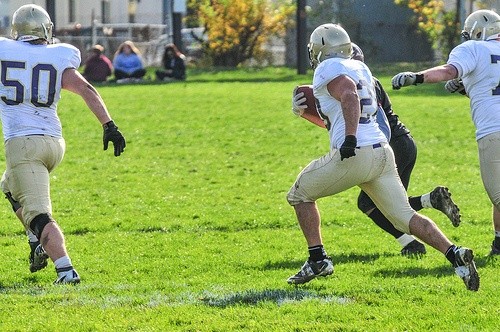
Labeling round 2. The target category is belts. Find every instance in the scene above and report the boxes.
[357,144,381,150]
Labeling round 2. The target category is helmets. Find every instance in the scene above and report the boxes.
[307,24,351,68]
[350,42,365,63]
[11,4,54,43]
[461,9,500,41]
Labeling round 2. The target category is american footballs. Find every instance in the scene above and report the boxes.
[296,85,320,117]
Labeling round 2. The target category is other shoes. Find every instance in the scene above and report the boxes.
[117,77,130,84]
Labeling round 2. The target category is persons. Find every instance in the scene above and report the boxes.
[155,43,185,81]
[84,45,112,82]
[113,41,146,83]
[287,24,481,291]
[0,4,126,283]
[350,42,462,255]
[392,10,500,255]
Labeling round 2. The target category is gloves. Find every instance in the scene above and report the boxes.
[102,120,126,156]
[391,71,423,90]
[339,136,357,162]
[292,87,308,116]
[444,78,466,96]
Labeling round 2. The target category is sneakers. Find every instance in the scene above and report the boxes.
[400,243,427,255]
[453,247,480,291]
[28,240,49,272]
[53,267,80,284]
[491,240,500,255]
[430,187,461,226]
[287,256,334,284]
